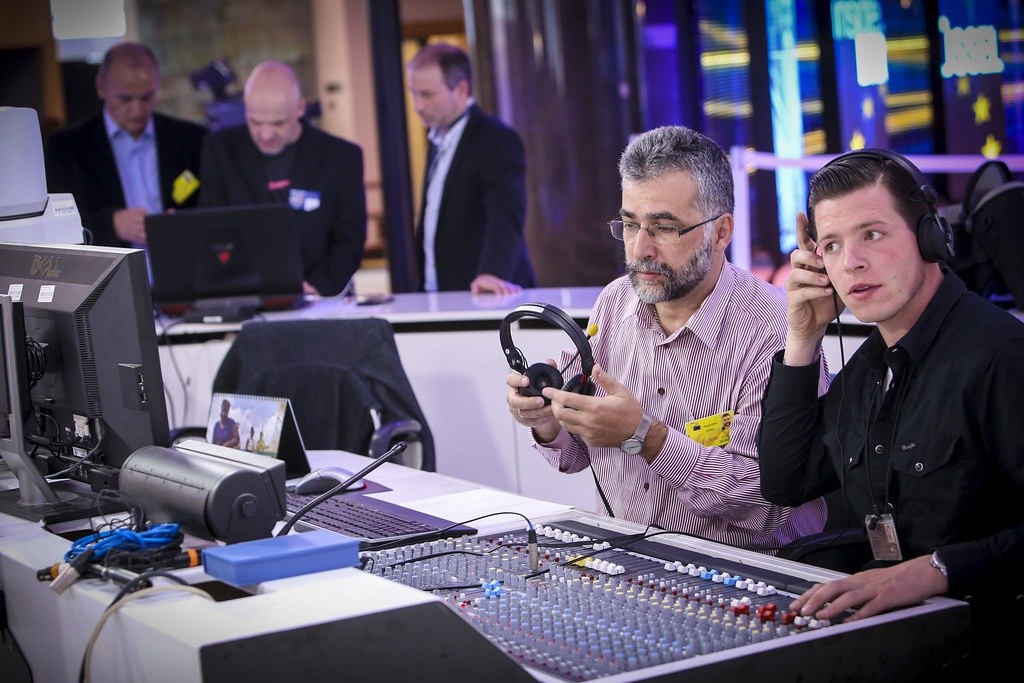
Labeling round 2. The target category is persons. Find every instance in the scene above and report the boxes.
[42,41,210,289]
[406,41,534,297]
[245,427,255,451]
[212,399,240,448]
[722,414,732,430]
[754,146,1024,621]
[886,525,894,542]
[196,60,367,296]
[504,126,830,557]
[256,432,268,451]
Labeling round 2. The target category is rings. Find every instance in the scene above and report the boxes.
[517,409,523,418]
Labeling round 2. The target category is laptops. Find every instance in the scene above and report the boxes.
[145,205,306,318]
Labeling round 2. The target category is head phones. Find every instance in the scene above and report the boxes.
[500,302,596,408]
[805,148,956,296]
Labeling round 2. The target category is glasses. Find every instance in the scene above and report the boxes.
[607,214,719,244]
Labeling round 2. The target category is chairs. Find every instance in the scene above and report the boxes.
[212,317,436,474]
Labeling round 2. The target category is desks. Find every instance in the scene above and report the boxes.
[305,451,587,538]
[155,287,876,513]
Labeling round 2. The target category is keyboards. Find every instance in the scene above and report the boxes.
[282,493,479,551]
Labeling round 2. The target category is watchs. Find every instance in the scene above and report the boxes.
[618,409,653,456]
[931,550,947,576]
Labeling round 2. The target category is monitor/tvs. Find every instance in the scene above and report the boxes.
[0,241,174,525]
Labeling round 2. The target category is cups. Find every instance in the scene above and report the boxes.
[354,267,392,304]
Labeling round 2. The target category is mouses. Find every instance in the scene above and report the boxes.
[295,466,366,495]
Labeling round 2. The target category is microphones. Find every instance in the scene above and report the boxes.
[276,441,407,538]
[560,324,598,375]
